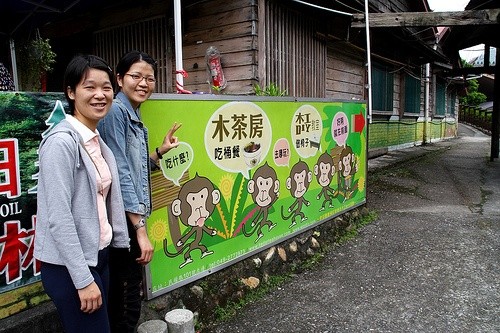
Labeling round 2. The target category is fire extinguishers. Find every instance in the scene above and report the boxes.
[206,46,228,90]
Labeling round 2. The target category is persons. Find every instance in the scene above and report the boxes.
[34,55,130,333]
[96,51,182,333]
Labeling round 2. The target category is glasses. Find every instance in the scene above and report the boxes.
[125,73,157,85]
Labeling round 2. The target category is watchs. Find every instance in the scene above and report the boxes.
[133,219,146,230]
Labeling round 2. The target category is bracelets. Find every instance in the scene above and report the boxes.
[155,147,163,159]
